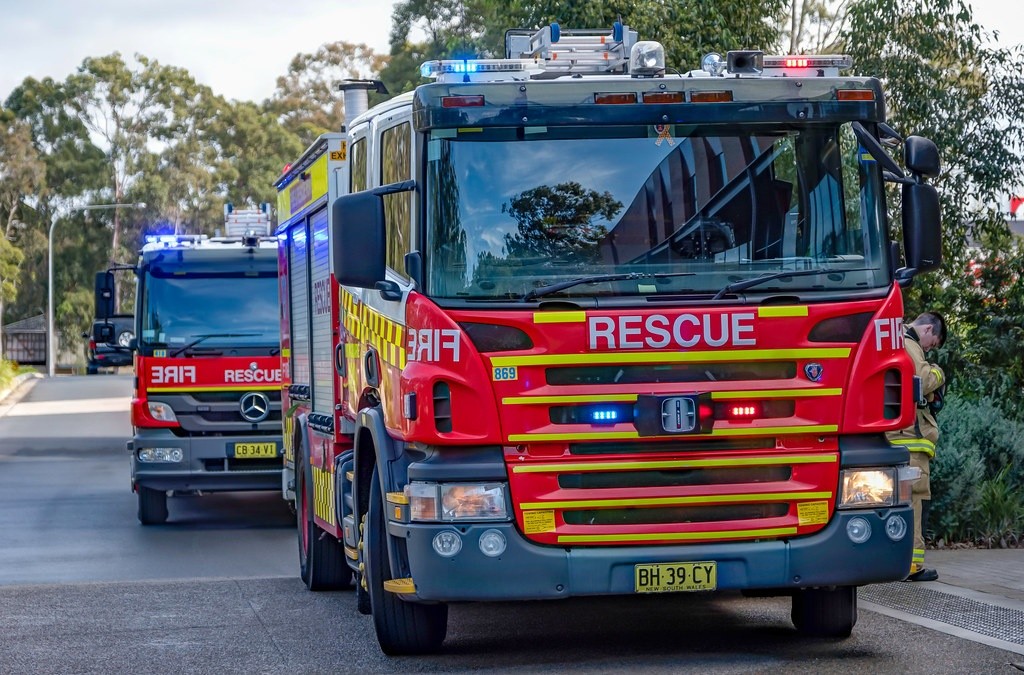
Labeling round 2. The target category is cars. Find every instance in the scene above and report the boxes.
[81,313,135,375]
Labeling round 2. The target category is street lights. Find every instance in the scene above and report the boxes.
[46,202,147,378]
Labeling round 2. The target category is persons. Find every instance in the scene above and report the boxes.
[903,311,954,582]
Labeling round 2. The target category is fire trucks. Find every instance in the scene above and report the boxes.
[95,201,283,525]
[271,20,943,656]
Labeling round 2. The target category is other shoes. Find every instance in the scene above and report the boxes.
[904,568,939,581]
